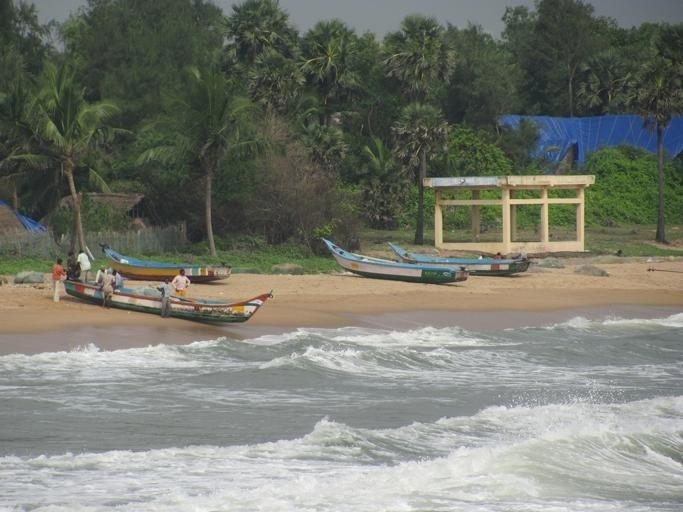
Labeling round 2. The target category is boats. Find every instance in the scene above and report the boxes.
[97,240,237,284]
[62,276,276,325]
[385,239,532,278]
[320,236,472,288]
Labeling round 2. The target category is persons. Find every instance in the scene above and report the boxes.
[171,269,190,297]
[156,277,172,318]
[51,249,121,309]
